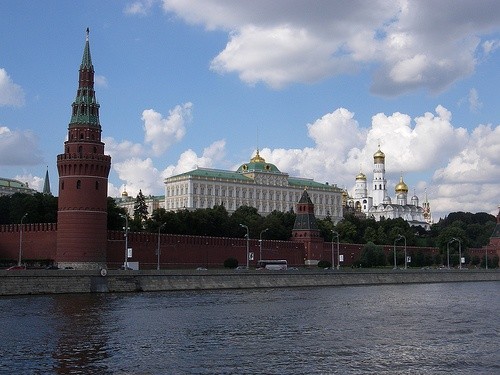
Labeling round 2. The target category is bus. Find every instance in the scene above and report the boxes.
[255,259,288,272]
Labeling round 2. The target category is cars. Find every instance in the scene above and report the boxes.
[233,266,248,270]
[196,267,208,271]
[288,266,298,271]
[421,264,448,270]
[59,266,75,270]
[44,266,61,270]
[4,265,24,270]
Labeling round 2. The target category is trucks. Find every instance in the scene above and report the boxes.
[121,261,139,270]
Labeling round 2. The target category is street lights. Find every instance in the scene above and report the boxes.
[240,223,249,268]
[331,230,340,270]
[157,222,167,270]
[260,228,269,260]
[394,234,407,270]
[19,213,28,266]
[118,213,127,270]
[447,239,455,268]
[452,237,462,270]
